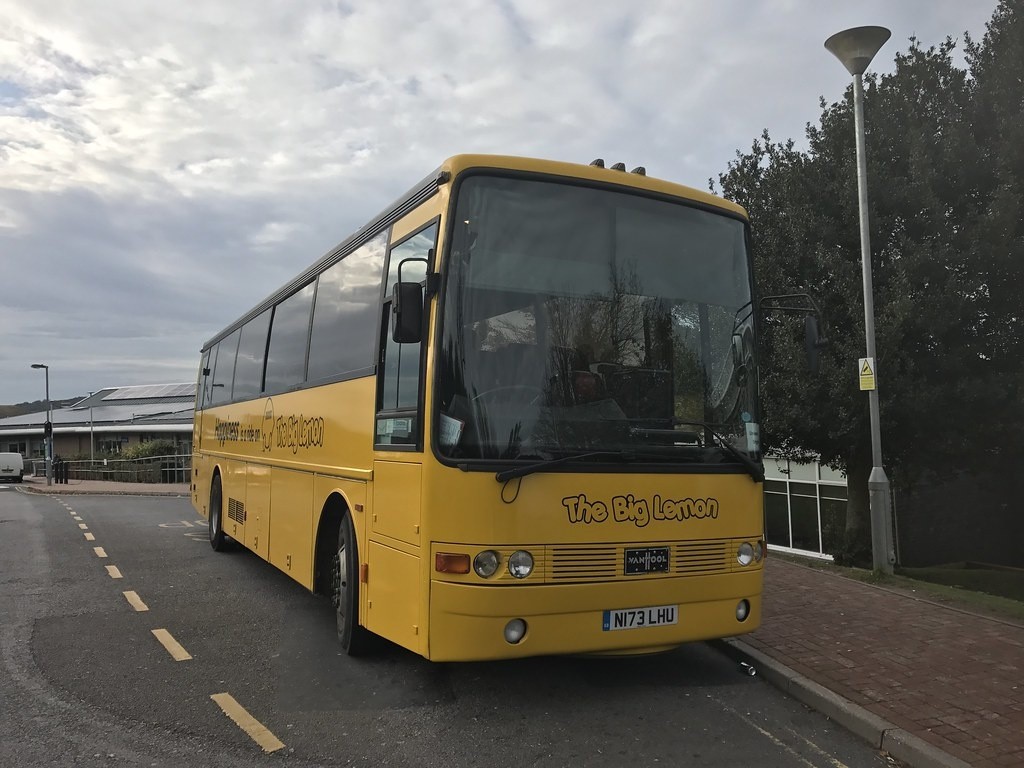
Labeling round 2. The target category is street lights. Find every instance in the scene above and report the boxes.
[822,23,900,578]
[31,363,52,486]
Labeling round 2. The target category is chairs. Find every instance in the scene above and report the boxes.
[440,328,626,406]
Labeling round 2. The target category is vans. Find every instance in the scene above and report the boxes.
[0,452,24,483]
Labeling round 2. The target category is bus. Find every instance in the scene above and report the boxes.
[189,152,831,670]
